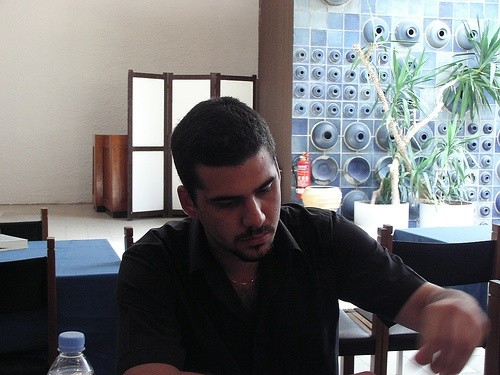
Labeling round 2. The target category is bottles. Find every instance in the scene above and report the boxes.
[47,331,95,375]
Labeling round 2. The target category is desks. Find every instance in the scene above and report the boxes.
[391,225,493,315]
[0,237,122,333]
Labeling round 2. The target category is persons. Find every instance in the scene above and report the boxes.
[108,95,491,375]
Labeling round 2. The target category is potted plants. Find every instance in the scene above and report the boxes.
[350,12,500,241]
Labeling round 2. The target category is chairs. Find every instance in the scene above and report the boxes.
[0,205,136,374]
[337,224,500,374]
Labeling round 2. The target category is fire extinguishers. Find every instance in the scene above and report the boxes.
[292,151,311,199]
